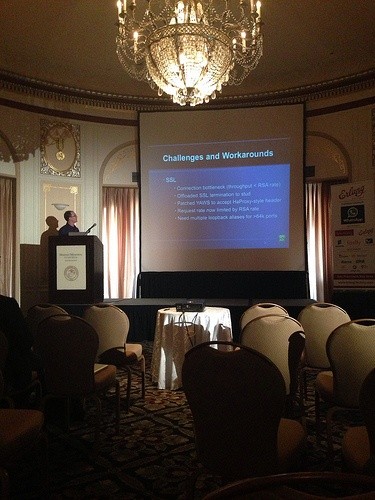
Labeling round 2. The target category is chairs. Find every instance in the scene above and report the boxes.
[0,294,146,500]
[182,300,375,500]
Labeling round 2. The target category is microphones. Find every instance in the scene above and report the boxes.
[83,223,96,236]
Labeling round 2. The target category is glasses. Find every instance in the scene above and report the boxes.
[72,215,77,218]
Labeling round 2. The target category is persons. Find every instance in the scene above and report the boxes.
[59,211,79,236]
[0,295,46,405]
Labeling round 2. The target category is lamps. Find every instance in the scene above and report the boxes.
[114,0,265,107]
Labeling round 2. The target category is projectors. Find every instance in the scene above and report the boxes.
[176,299,206,312]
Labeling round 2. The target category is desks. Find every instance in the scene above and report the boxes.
[149,306,233,391]
